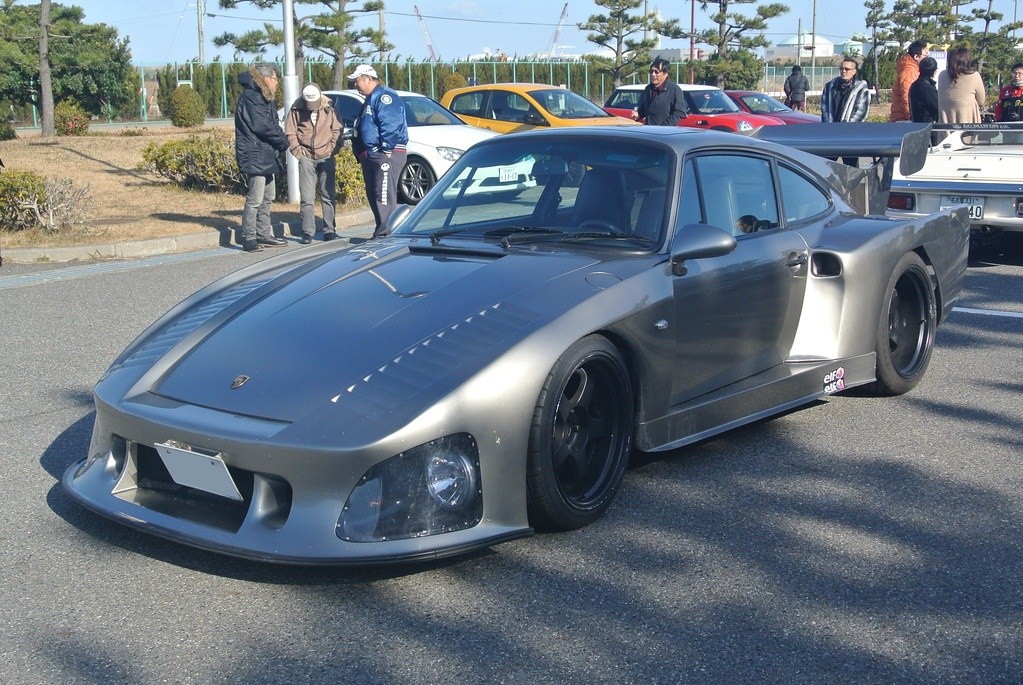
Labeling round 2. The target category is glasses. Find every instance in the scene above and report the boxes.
[1011,71,1023,74]
[649,69,661,73]
[841,67,852,70]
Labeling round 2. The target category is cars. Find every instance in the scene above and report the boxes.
[603,84,786,135]
[277,88,537,205]
[691,91,823,125]
[419,83,646,186]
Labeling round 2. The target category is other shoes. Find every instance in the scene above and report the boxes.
[324,232,340,241]
[302,232,312,243]
[367,236,379,242]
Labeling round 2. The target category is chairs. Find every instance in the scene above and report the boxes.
[568,165,740,237]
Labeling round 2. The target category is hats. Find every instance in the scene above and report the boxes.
[347,63,378,80]
[302,81,321,110]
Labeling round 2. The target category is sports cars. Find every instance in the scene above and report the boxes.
[57,124,972,567]
[886,120,1022,236]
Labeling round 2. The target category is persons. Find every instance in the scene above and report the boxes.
[784,65,809,113]
[890,39,928,121]
[937,46,985,147]
[994,62,1023,129]
[908,56,938,148]
[636,54,687,129]
[285,83,343,242]
[820,58,870,168]
[346,64,408,241]
[235,66,290,252]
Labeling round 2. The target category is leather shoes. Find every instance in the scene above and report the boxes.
[242,239,263,252]
[257,235,288,246]
